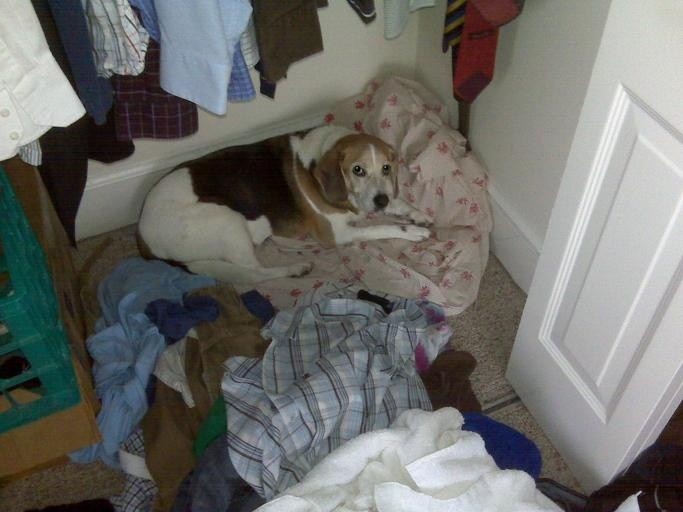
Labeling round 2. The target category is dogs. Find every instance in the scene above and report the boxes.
[134,124,437,285]
